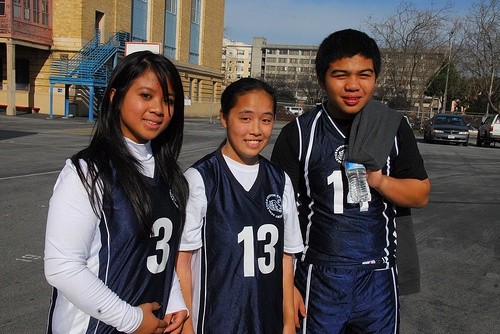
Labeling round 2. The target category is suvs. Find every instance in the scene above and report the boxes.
[476,114,500,147]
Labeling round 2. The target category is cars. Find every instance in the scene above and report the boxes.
[423,113,470,147]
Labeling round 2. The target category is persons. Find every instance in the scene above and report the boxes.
[43,50,190,334]
[270,28,431,334]
[174,77,305,334]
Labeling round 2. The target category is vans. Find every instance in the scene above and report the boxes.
[287,105,303,115]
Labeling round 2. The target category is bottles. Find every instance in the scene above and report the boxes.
[345,161,372,203]
